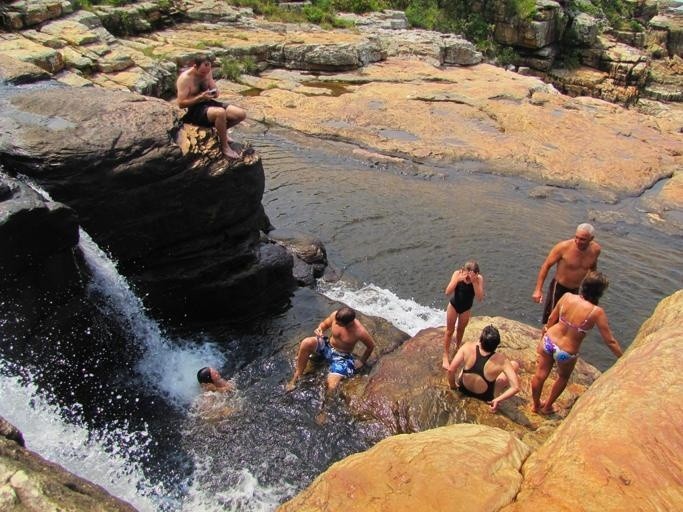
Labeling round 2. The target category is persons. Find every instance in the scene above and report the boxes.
[441,259,484,369]
[176,55,246,160]
[531,271,623,414]
[448,324,518,414]
[195,367,236,417]
[286,306,375,424]
[530,223,601,353]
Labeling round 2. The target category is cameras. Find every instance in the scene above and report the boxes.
[208,89,216,95]
